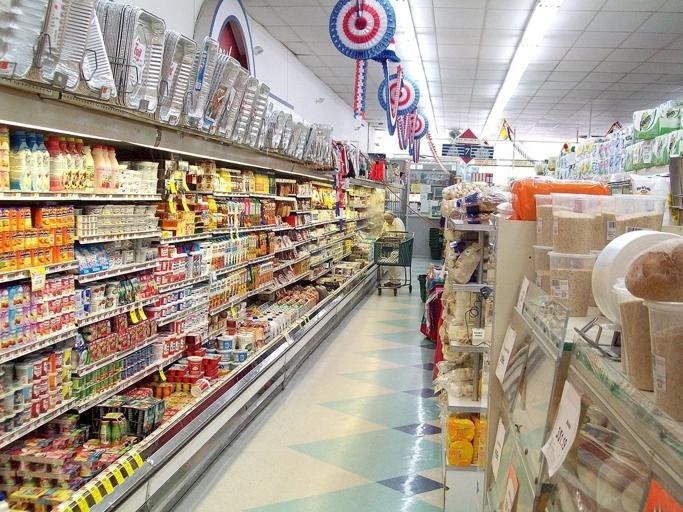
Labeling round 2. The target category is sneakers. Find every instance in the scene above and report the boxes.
[389,284,401,288]
[384,282,391,287]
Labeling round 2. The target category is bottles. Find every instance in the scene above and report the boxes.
[97,411,128,448]
[471,172,493,184]
[11,127,121,192]
[252,296,309,337]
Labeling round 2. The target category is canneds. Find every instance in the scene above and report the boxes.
[0,205,75,272]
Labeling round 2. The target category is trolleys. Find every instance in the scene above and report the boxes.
[372,229,416,296]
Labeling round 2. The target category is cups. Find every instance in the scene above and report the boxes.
[186,354,202,375]
[189,378,210,397]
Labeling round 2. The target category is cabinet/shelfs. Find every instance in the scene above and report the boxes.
[424,215,683,512]
[0,73,411,511]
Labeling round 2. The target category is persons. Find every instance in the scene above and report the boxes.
[382,210,406,288]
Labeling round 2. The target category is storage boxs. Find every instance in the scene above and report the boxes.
[536,97,683,184]
